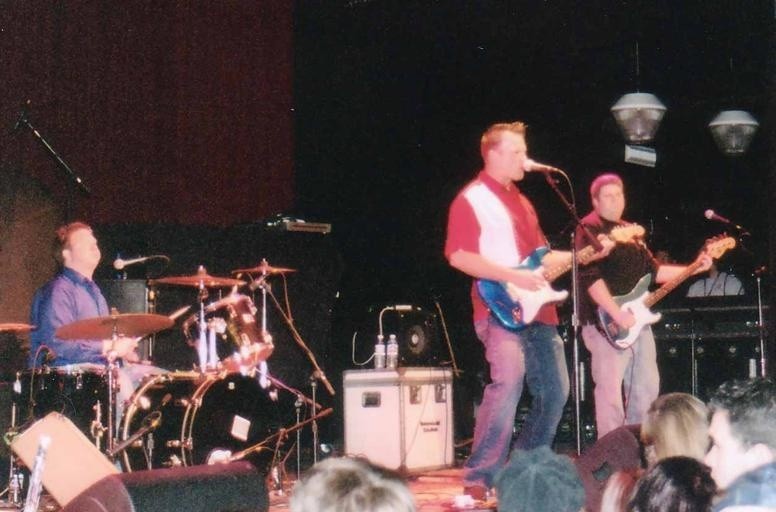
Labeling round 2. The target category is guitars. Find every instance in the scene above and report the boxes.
[475,223,645,329]
[599,233,736,350]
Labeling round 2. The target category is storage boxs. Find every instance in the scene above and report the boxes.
[339,366,459,478]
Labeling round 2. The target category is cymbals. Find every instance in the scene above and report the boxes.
[56,314,175,339]
[155,276,247,288]
[0,322,36,332]
[231,267,295,273]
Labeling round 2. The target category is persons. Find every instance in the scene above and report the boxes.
[624,455,717,511]
[685,263,747,297]
[570,171,713,441]
[704,376,775,512]
[287,449,415,511]
[26,218,144,366]
[640,391,712,464]
[493,444,589,511]
[442,117,615,492]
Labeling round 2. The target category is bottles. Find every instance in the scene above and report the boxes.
[373,333,386,369]
[9,466,23,505]
[387,333,400,367]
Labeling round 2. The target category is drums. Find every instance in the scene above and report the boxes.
[18,365,116,458]
[182,296,275,377]
[120,372,282,475]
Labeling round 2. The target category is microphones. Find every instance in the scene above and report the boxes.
[704,208,740,229]
[522,159,559,173]
[113,256,150,269]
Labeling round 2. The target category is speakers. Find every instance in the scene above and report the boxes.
[60,461,269,512]
[368,304,447,365]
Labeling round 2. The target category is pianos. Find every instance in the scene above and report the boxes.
[650,294,776,339]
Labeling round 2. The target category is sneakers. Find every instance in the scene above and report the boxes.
[464,486,488,503]
